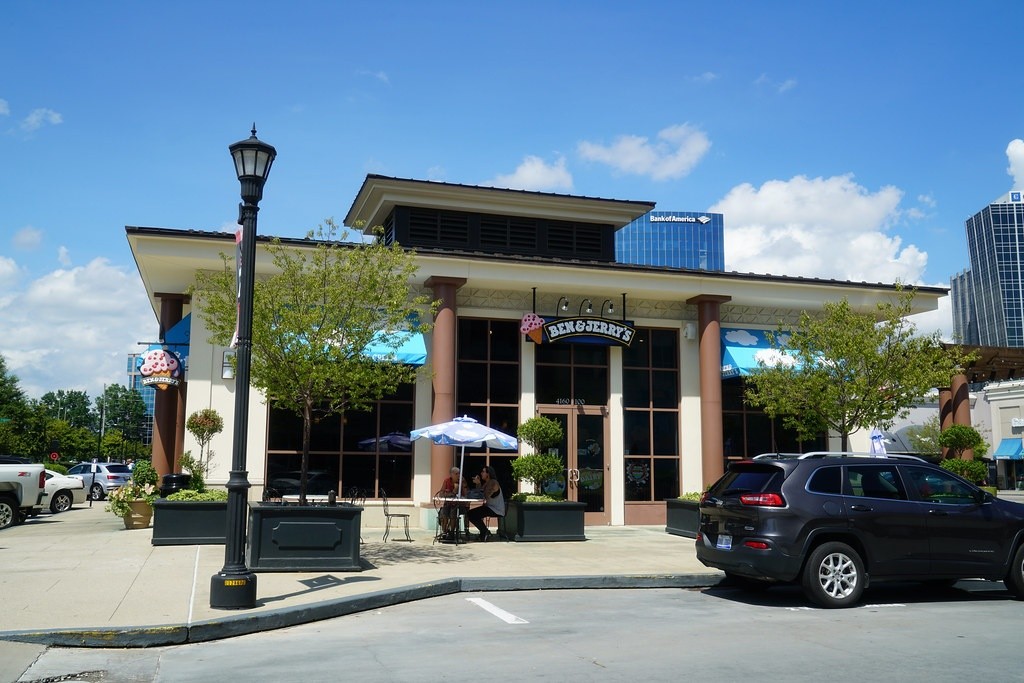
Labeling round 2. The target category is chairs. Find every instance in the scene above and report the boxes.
[264,486,367,545]
[382,489,413,542]
[432,490,464,547]
[485,486,513,541]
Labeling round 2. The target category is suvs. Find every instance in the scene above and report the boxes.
[0,455,27,464]
[695,451,1024,609]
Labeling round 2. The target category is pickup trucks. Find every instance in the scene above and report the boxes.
[0,463,49,531]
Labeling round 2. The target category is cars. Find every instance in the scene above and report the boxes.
[65,462,132,500]
[926,475,943,484]
[30,469,88,514]
[272,471,328,489]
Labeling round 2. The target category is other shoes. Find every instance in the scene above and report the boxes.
[480,530,490,541]
[441,529,454,540]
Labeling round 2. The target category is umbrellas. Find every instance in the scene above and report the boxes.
[361,431,410,449]
[870,424,886,457]
[408,413,518,500]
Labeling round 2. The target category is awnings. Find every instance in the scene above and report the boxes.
[137,312,190,374]
[993,437,1023,461]
[720,327,853,383]
[269,312,427,368]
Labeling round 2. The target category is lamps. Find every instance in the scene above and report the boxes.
[223,350,237,380]
[557,296,570,319]
[579,299,592,317]
[601,300,614,319]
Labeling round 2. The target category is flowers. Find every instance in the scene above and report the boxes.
[104,481,162,519]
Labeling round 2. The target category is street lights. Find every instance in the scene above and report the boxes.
[209,122,279,610]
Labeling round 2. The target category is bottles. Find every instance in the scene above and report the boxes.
[445,489,451,497]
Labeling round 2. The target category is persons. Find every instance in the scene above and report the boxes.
[466,466,507,542]
[127,458,136,471]
[437,466,473,541]
[911,472,952,494]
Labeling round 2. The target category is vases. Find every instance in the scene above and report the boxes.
[123,497,155,529]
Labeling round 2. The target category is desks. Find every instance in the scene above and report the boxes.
[433,497,482,540]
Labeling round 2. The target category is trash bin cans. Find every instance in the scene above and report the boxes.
[160,474,191,498]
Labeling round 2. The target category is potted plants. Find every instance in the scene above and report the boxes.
[938,425,998,497]
[504,418,589,542]
[150,487,229,546]
[665,492,702,539]
